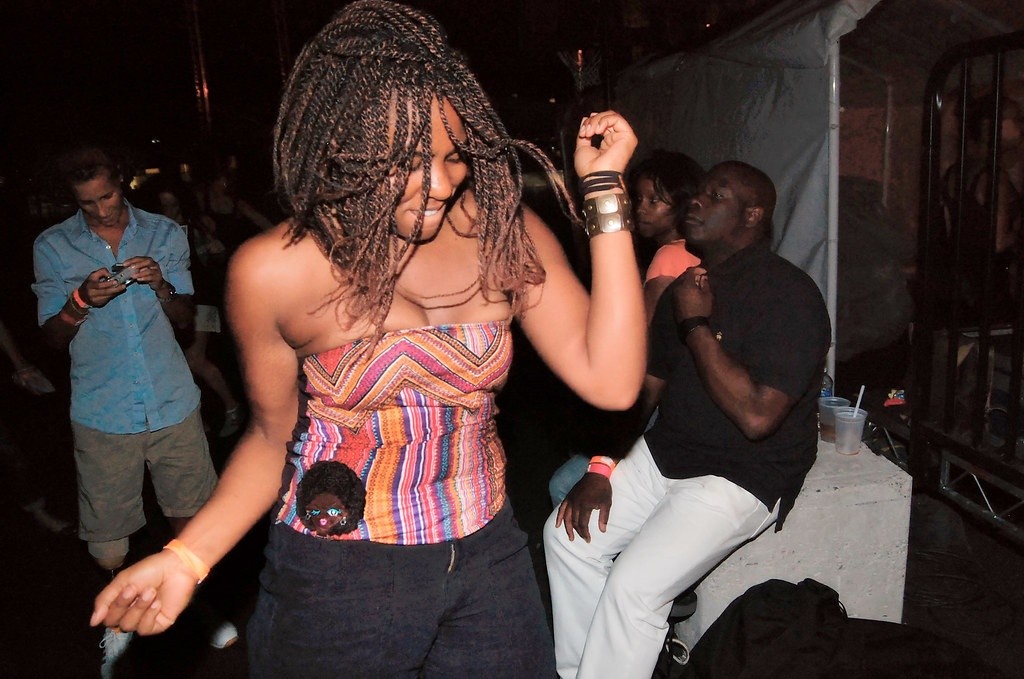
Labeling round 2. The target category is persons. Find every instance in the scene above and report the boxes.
[537,147,708,510]
[90,0,648,679]
[542,161,831,679]
[0,147,275,571]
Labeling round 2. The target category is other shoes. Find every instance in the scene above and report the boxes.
[218,404,245,438]
[187,601,239,650]
[11,366,56,396]
[99,626,137,679]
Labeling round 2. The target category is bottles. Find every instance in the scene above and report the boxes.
[816,367,833,431]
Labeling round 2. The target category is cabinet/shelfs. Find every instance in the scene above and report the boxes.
[673,416,913,655]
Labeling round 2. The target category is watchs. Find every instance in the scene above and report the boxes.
[154,282,177,303]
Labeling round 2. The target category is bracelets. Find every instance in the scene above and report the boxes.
[578,170,635,239]
[60,309,87,327]
[70,296,89,315]
[677,316,709,345]
[163,539,210,584]
[73,287,90,309]
[587,456,616,479]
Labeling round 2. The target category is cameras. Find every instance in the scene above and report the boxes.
[107,263,140,285]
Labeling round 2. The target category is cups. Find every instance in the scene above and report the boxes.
[832,407,868,455]
[818,397,851,443]
